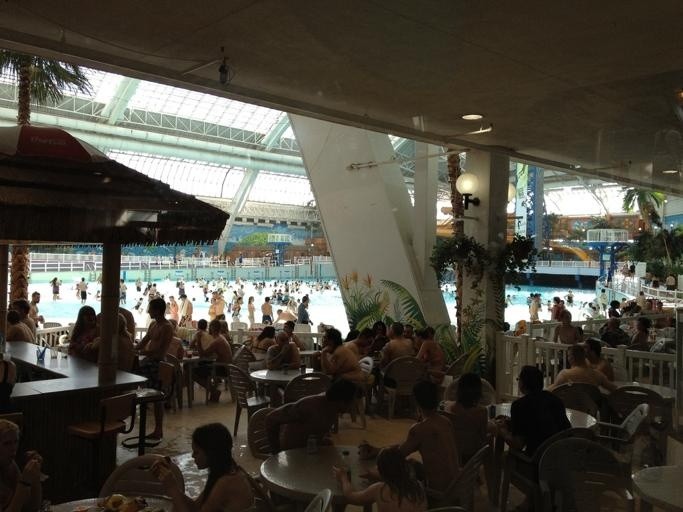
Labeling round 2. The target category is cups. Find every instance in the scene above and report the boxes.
[36,348,44,361]
[49,347,59,359]
[186,351,192,359]
[60,345,67,358]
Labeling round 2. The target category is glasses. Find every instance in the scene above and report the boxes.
[280,336,290,343]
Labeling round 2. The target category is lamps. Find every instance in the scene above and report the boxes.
[452,171,480,213]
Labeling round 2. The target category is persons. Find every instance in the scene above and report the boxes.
[0,253,339,512]
[307,266,681,512]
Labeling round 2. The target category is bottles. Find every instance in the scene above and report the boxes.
[299,364,306,375]
[487,405,495,418]
[281,364,288,374]
[340,450,350,485]
